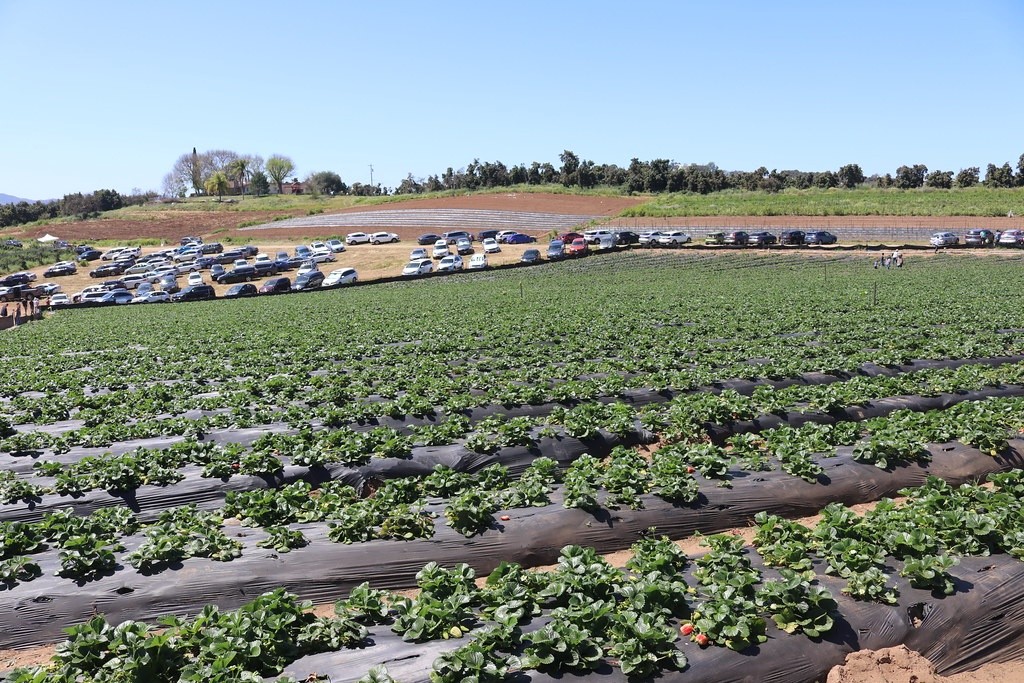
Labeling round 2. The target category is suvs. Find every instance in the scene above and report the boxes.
[370,232,399,245]
[346,232,373,245]
[171,285,216,302]
[131,291,171,303]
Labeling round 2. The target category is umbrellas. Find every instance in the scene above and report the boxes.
[38,234,59,244]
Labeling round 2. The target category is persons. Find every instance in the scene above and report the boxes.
[933,237,941,254]
[994,229,1001,247]
[46,297,50,305]
[22,298,27,312]
[1,303,8,316]
[892,249,899,264]
[886,256,891,269]
[873,261,878,269]
[34,298,39,306]
[15,303,21,317]
[980,230,986,244]
[895,254,903,268]
[29,300,34,315]
[881,253,884,266]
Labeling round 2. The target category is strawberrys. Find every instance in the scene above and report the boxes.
[695,633,708,645]
[442,626,470,639]
[680,623,694,636]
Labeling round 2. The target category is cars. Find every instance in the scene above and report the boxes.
[47,261,77,268]
[310,241,329,252]
[50,294,70,304]
[294,245,312,257]
[326,240,344,253]
[134,283,158,297]
[0,271,61,302]
[71,284,110,303]
[5,240,23,248]
[929,228,1024,248]
[158,276,179,294]
[99,281,127,291]
[93,291,134,304]
[53,241,93,254]
[259,277,291,296]
[75,251,103,263]
[89,236,335,286]
[290,271,331,290]
[400,224,836,275]
[322,267,359,288]
[223,283,260,297]
[43,266,78,277]
